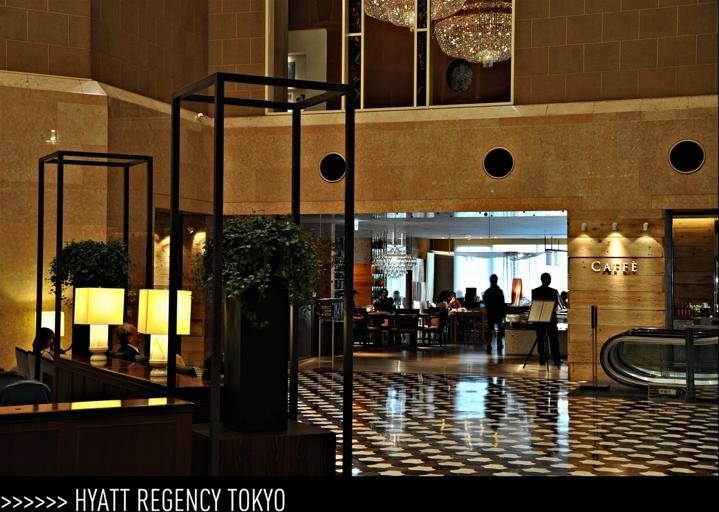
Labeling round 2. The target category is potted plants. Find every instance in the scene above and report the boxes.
[188,201,342,434]
[45,236,133,362]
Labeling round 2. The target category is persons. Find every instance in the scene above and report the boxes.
[372,272,568,365]
[116,322,140,354]
[33,327,66,361]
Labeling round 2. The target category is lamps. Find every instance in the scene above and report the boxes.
[372,213,418,279]
[611,220,619,232]
[362,1,414,29]
[579,219,587,232]
[642,220,648,232]
[431,1,513,68]
[135,285,192,378]
[73,285,126,362]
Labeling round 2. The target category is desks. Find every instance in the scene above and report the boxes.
[317,296,568,364]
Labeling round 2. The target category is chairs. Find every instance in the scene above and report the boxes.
[0,380,52,407]
